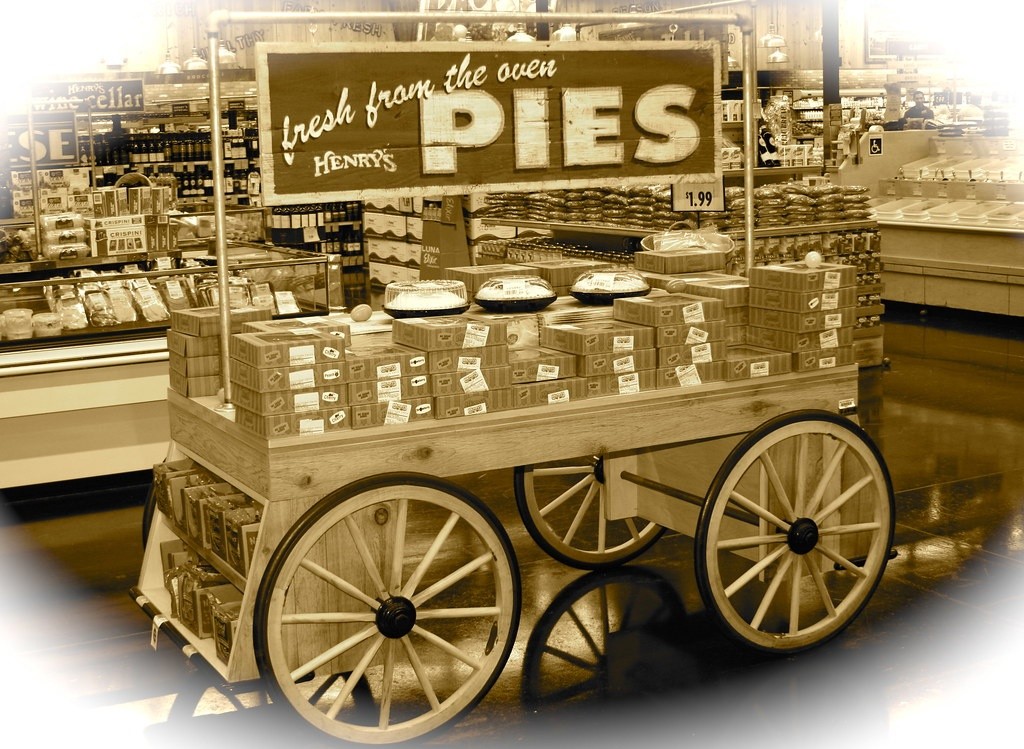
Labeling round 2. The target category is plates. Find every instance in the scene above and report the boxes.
[568,284,651,303]
[472,294,557,314]
[381,305,470,319]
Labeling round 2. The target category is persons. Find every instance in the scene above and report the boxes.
[904,91,934,119]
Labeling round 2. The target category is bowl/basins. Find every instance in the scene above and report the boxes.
[3,309,62,339]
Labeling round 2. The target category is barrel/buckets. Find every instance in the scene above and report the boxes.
[641,222,734,274]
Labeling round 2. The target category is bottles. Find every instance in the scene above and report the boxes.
[143,165,176,210]
[97,140,104,166]
[315,243,326,253]
[180,133,212,162]
[120,138,128,164]
[343,267,364,285]
[177,163,214,196]
[759,121,781,166]
[111,139,120,165]
[272,205,324,242]
[793,97,887,119]
[758,134,775,166]
[224,167,247,194]
[248,162,260,196]
[222,129,259,159]
[324,202,359,223]
[128,136,179,164]
[326,232,361,255]
[89,146,97,166]
[104,140,111,165]
[80,144,89,166]
